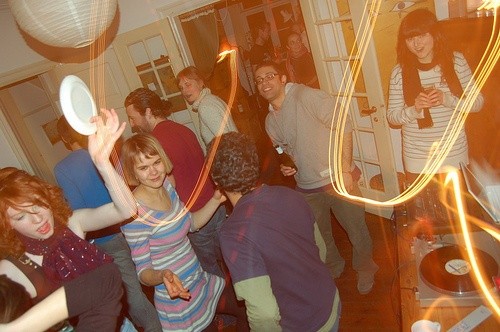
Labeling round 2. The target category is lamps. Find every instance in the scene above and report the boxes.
[216,7,233,55]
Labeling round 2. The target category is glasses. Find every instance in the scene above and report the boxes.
[254,72,280,84]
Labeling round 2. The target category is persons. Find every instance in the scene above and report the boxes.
[385,10,484,185]
[0,18,378,332]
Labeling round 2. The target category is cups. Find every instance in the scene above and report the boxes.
[410,319,440,332]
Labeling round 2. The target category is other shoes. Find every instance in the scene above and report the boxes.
[357,269,376,294]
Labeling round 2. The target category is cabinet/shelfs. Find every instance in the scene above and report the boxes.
[435,12,500,176]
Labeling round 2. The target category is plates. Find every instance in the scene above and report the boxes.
[59,75,98,135]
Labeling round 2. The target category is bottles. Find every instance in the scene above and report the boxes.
[275,145,298,175]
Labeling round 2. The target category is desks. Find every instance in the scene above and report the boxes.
[395,203,500,332]
